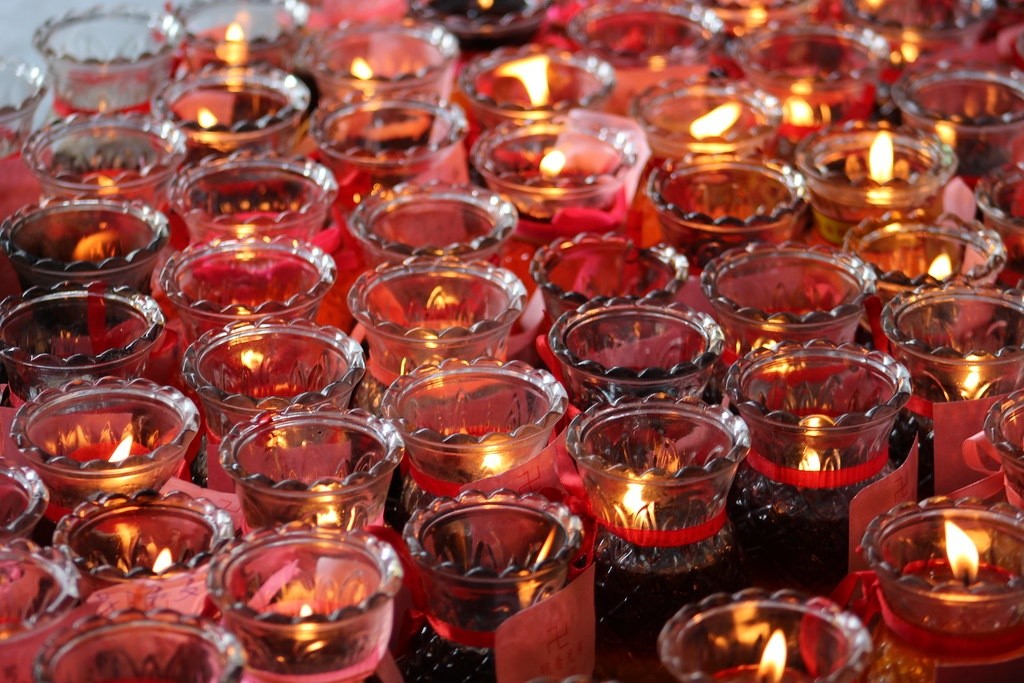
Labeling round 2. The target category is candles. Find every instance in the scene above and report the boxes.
[0,0,1024,683]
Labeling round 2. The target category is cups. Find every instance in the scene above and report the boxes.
[0,1,1024,683]
